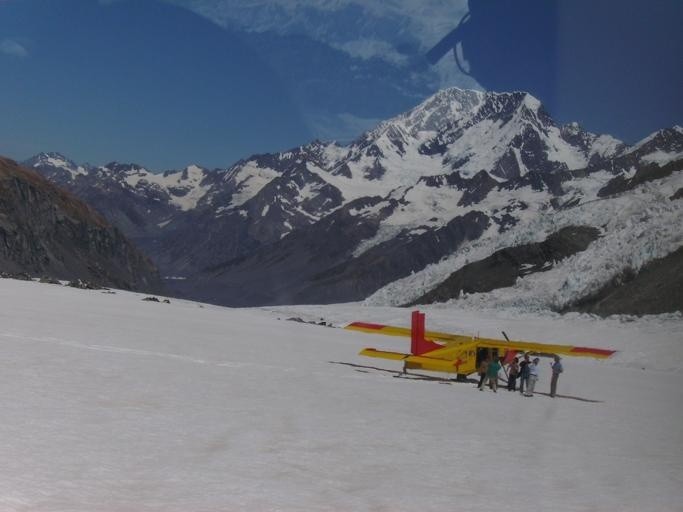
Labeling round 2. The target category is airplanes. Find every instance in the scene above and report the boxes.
[339,308,620,383]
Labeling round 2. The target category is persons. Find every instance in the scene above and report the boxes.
[476,352,564,398]
